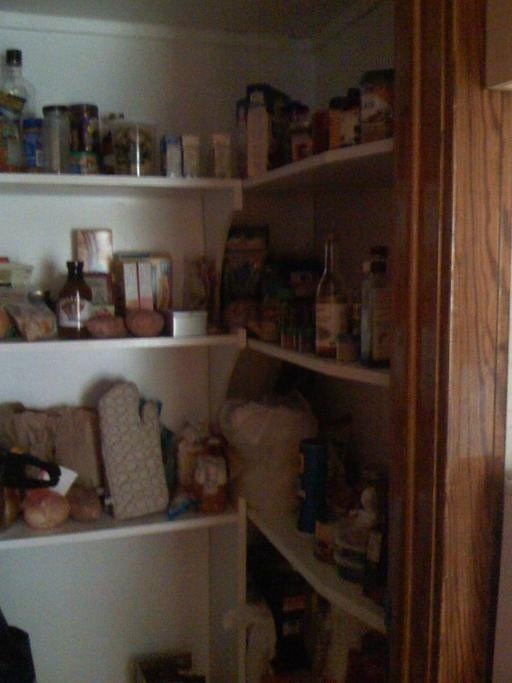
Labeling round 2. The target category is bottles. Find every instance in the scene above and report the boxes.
[1,48,39,122]
[0,257,12,286]
[42,104,74,172]
[314,228,348,356]
[360,245,394,364]
[339,86,362,146]
[192,436,229,513]
[58,260,93,339]
[23,117,44,173]
[101,111,126,173]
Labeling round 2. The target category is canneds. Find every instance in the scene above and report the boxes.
[23,103,100,175]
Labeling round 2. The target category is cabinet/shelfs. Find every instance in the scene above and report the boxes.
[233,128,401,679]
[3,157,250,683]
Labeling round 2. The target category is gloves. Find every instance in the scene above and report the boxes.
[97,380,169,520]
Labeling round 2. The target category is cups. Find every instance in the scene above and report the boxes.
[111,119,156,175]
[312,112,330,155]
[296,436,328,530]
